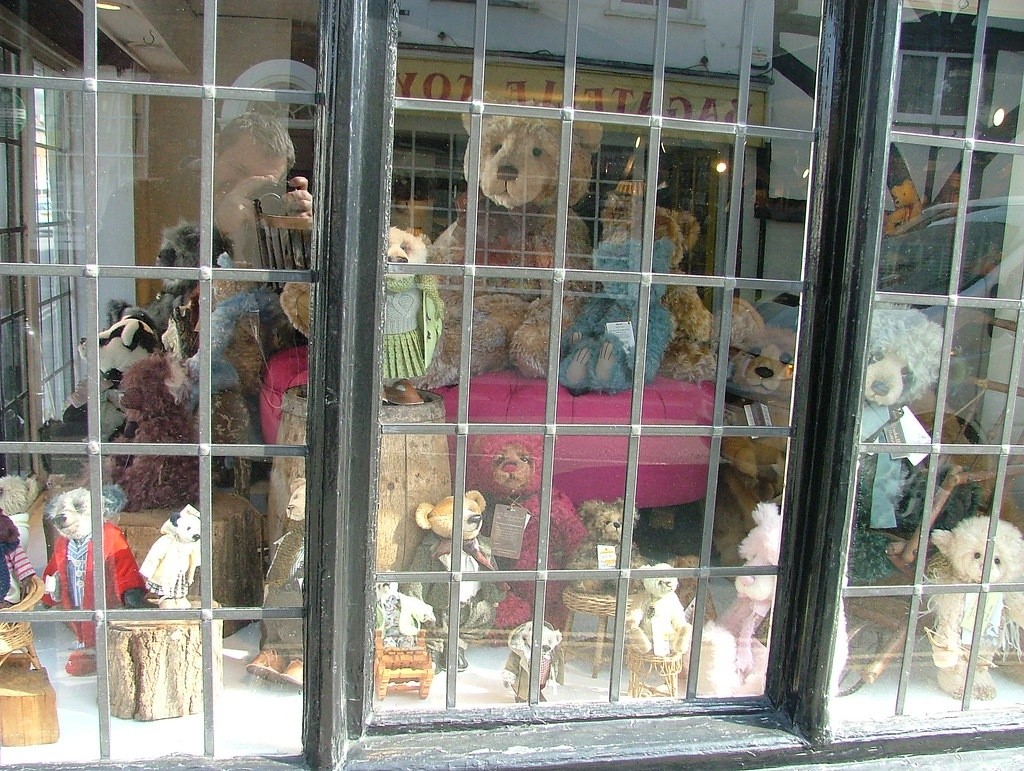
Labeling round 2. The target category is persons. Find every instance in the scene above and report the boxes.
[98,113,313,332]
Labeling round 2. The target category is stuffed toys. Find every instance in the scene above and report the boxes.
[246,90,1024,704]
[0,218,270,676]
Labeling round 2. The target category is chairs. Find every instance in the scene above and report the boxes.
[374,628,435,701]
[251,199,313,269]
[836,411,992,683]
[1,574,44,673]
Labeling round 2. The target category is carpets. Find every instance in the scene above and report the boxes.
[634,502,721,561]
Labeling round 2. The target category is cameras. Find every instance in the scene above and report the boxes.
[249,181,296,217]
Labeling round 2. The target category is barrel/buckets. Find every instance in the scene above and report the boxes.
[266,385,453,587]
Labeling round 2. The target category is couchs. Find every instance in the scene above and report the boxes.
[260,343,716,530]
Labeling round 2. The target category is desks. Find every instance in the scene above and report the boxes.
[562,585,637,679]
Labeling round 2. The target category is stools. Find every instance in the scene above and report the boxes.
[628,646,683,699]
